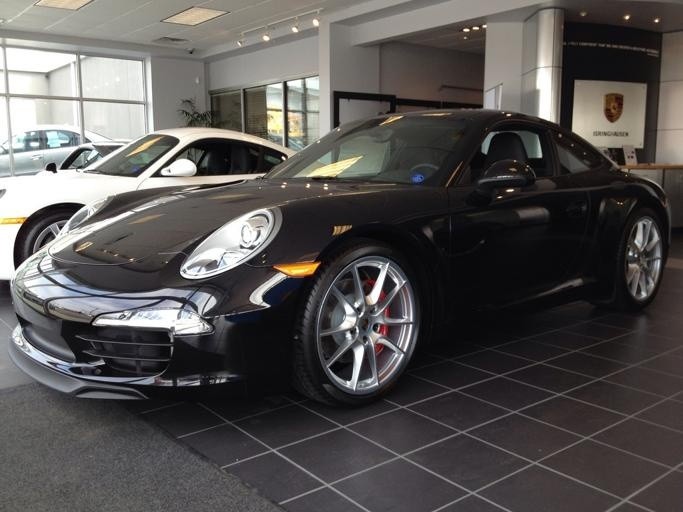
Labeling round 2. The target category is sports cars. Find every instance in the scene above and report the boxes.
[0,125,298,283]
[6,105,674,415]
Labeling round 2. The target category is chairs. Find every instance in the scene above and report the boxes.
[476,132,531,184]
[206,143,253,174]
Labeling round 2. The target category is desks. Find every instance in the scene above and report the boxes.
[617,163,683,188]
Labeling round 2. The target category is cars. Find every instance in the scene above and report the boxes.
[0,123,113,193]
[37,138,134,175]
